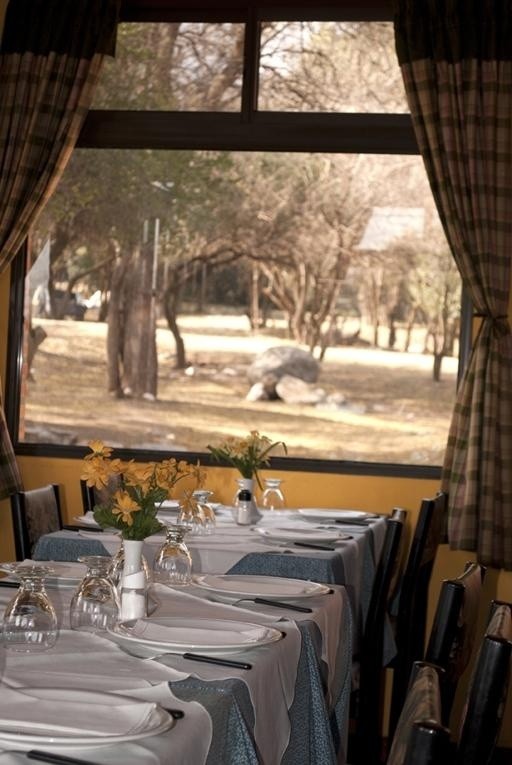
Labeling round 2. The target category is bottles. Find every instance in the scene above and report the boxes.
[236,489,252,526]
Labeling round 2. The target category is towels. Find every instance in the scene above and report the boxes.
[202,575,322,596]
[122,619,268,644]
[0,683,157,738]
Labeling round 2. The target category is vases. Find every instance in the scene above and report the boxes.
[234,480,261,524]
[117,540,158,619]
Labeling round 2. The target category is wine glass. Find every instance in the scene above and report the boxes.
[152,523,192,588]
[68,554,121,632]
[258,477,285,520]
[2,566,58,654]
[188,489,217,537]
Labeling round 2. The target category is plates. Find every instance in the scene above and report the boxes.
[104,615,282,653]
[187,572,332,603]
[253,526,350,544]
[296,506,380,521]
[0,687,177,747]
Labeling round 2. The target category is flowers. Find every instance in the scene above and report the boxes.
[80,440,207,541]
[207,430,289,492]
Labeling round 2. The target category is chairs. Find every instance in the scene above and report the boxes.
[81,473,124,515]
[10,482,69,561]
